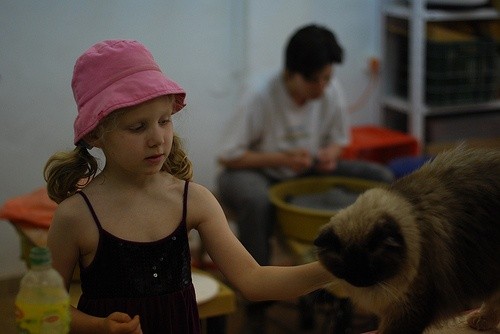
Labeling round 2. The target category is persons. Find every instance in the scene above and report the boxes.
[47,38,336,334]
[218,24,395,334]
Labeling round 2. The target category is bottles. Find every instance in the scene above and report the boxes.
[14,245,71,334]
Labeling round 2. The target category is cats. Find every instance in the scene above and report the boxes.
[311,142,500,334]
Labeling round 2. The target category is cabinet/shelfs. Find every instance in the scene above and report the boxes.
[382,0,500,152]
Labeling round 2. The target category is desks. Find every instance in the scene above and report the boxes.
[0,267,236,334]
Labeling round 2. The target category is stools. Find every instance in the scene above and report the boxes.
[339,123,418,165]
[270,235,351,334]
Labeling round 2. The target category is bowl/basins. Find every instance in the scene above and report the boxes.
[267,176,390,242]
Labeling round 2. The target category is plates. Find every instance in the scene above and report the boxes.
[191,271,219,304]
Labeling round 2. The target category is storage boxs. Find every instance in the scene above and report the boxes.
[398,34,499,102]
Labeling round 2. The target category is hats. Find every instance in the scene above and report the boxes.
[71,40,188,145]
